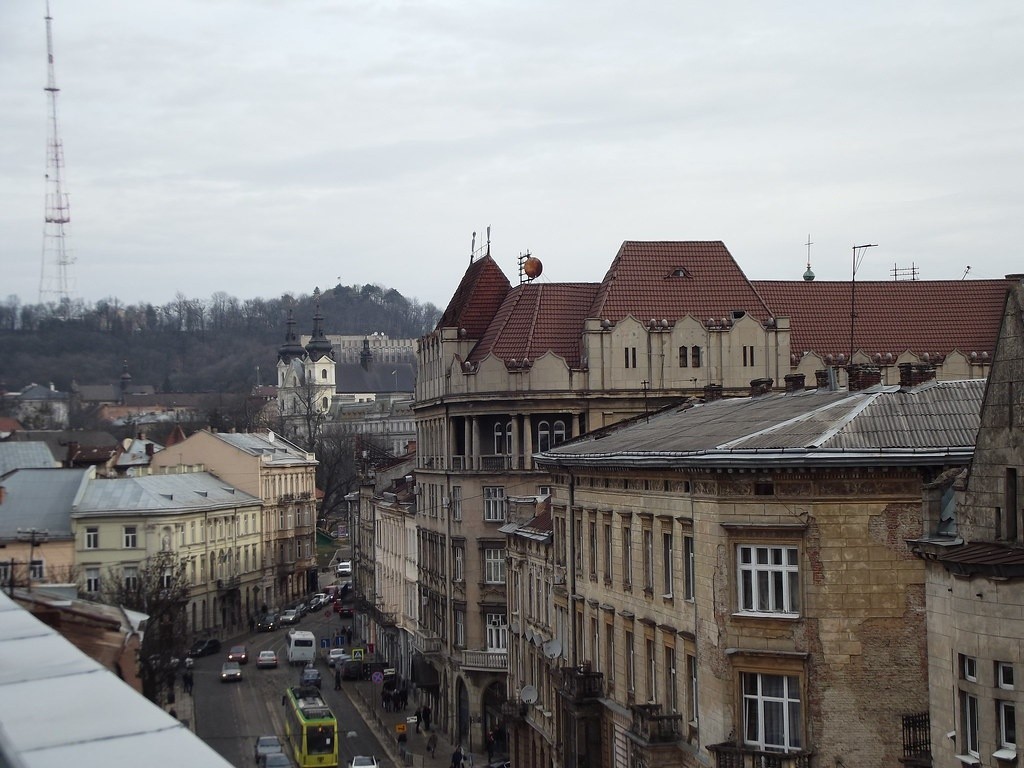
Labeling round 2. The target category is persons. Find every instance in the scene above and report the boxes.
[380,680,408,712]
[247,617,255,631]
[422,705,430,731]
[332,625,353,646]
[427,730,437,759]
[169,707,177,718]
[260,603,267,619]
[451,744,466,768]
[182,671,194,693]
[414,708,422,733]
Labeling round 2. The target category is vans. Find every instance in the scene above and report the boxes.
[285,626,316,664]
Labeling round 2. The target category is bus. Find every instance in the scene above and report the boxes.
[282,684,338,768]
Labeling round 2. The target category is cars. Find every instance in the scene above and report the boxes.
[347,755,381,768]
[300,669,321,689]
[188,638,221,658]
[327,648,360,680]
[256,650,277,669]
[227,645,249,665]
[258,580,353,632]
[219,661,242,682]
[255,736,294,768]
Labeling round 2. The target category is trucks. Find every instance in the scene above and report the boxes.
[337,560,352,576]
